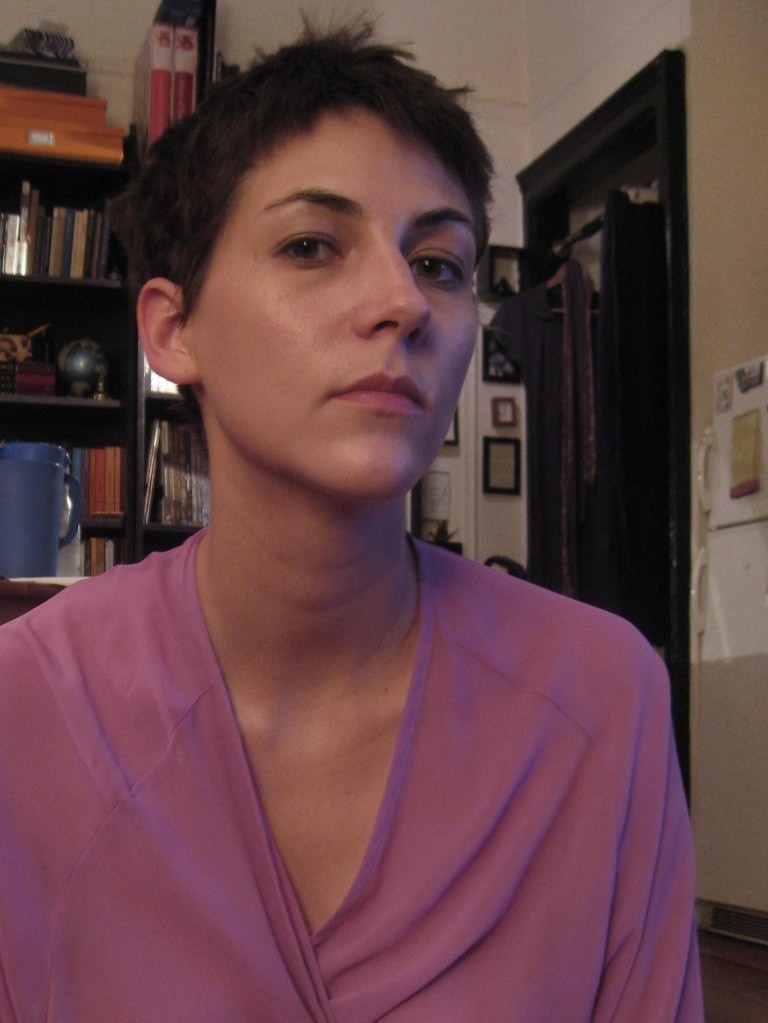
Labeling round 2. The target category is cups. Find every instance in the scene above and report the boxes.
[0,443,82,578]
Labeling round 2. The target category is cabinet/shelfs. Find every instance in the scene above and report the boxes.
[0,0,227,579]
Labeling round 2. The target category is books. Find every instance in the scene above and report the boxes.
[144,419,209,525]
[133,25,198,148]
[70,447,129,577]
[0,180,124,280]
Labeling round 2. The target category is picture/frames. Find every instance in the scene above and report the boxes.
[482,330,522,385]
[491,397,517,428]
[481,435,522,497]
[476,243,524,303]
[444,402,460,447]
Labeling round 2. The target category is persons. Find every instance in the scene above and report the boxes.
[0,25,703,1023]
